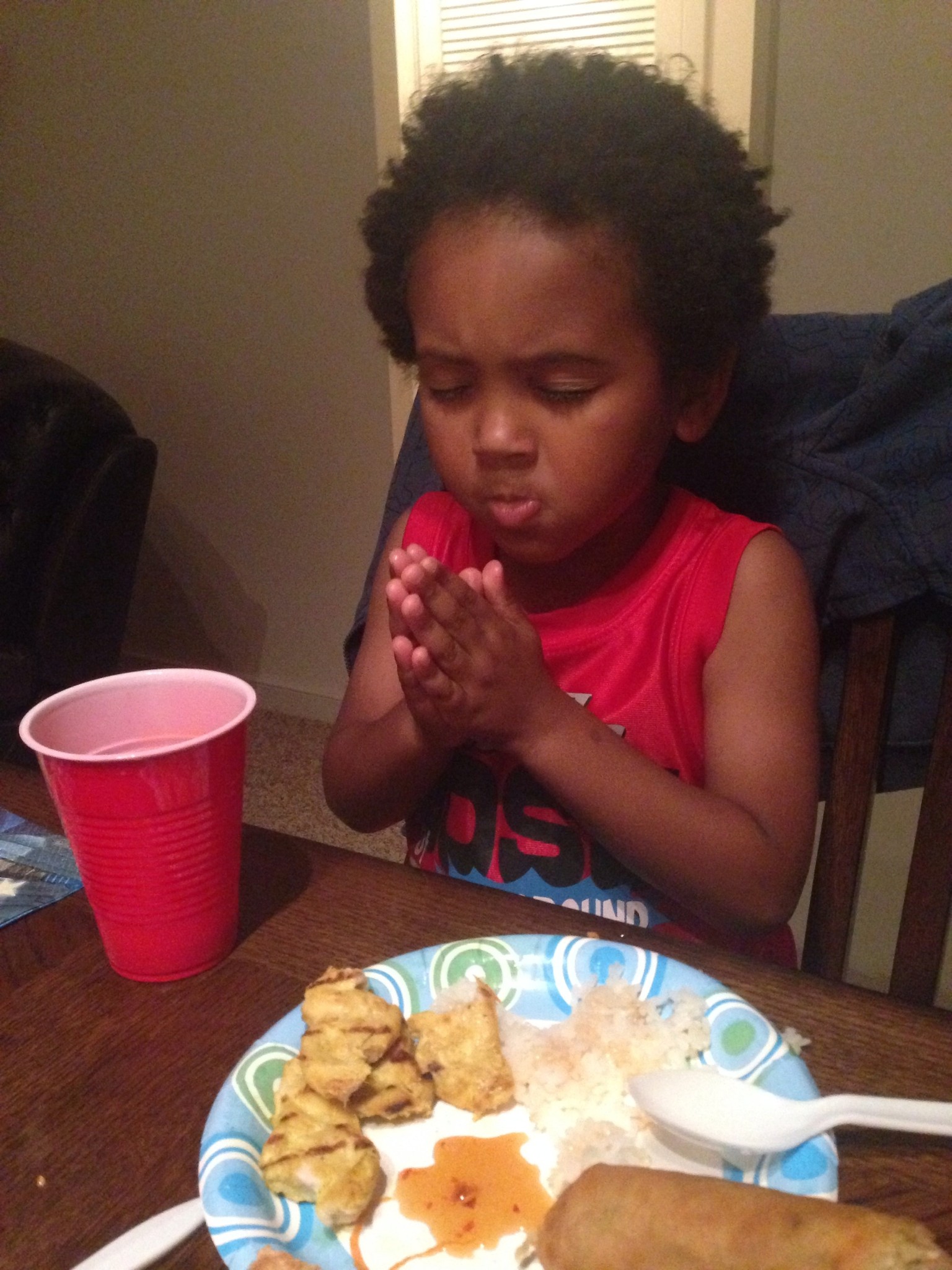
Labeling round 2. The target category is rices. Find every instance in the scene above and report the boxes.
[434,979,814,1197]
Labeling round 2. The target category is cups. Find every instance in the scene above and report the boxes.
[18,667,257,981]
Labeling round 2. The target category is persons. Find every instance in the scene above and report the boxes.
[321,40,816,969]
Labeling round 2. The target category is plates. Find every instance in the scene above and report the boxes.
[197,932,839,1270]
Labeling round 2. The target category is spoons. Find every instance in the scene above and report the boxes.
[628,1067,951,1152]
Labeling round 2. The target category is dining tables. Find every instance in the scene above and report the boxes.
[0,755,952,1265]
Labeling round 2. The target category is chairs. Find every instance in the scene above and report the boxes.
[346,301,948,1018]
